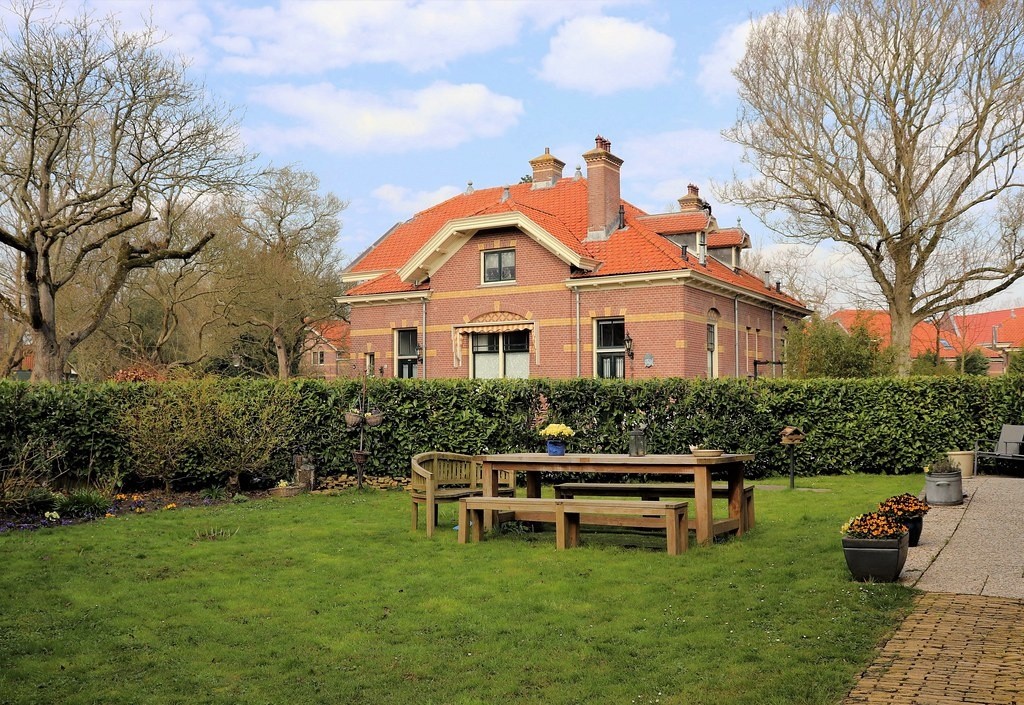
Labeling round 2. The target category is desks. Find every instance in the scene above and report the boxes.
[475,451,752,545]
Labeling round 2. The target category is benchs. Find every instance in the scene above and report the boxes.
[458,482,756,554]
[411,451,516,537]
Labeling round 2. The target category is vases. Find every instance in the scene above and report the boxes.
[346,413,361,427]
[367,413,384,427]
[902,514,924,546]
[352,450,371,463]
[546,439,563,455]
[949,450,974,480]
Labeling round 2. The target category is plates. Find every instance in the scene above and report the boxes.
[691,449,724,457]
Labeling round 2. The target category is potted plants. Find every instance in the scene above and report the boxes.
[925,452,963,506]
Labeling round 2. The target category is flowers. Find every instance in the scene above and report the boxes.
[347,408,364,416]
[881,492,930,517]
[843,532,908,582]
[364,410,381,418]
[844,514,910,540]
[539,423,576,440]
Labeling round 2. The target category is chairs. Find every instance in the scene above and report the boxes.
[974,426,1024,476]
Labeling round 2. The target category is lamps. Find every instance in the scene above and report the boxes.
[379,366,383,375]
[415,344,424,363]
[624,334,634,358]
